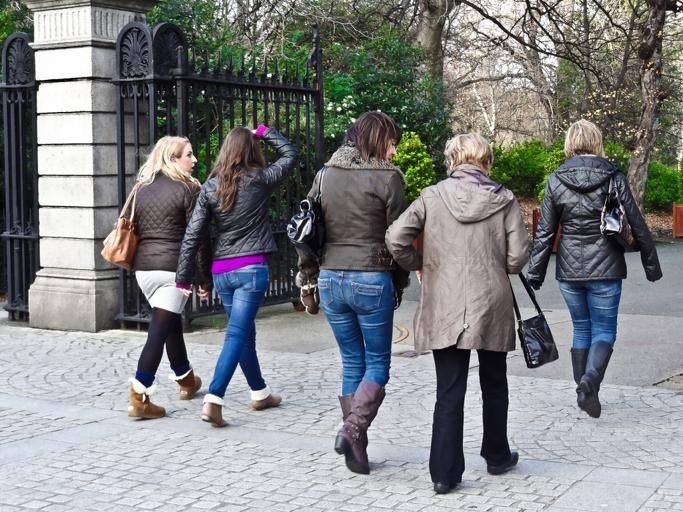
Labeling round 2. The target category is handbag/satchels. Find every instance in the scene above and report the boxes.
[519,315,559,368]
[599,175,640,252]
[286,166,324,246]
[98,184,138,271]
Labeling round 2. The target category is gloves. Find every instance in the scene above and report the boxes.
[300,284,320,316]
[394,285,404,308]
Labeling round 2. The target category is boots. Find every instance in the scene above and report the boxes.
[169,369,202,400]
[333,381,386,474]
[127,376,166,418]
[200,393,229,427]
[570,341,614,418]
[250,385,281,410]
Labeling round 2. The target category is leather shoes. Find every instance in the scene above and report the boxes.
[488,452,518,474]
[434,481,450,494]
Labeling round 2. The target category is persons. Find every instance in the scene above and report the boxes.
[528,119,662,419]
[294,111,412,475]
[384,132,529,492]
[119,136,211,419]
[173,125,299,427]
